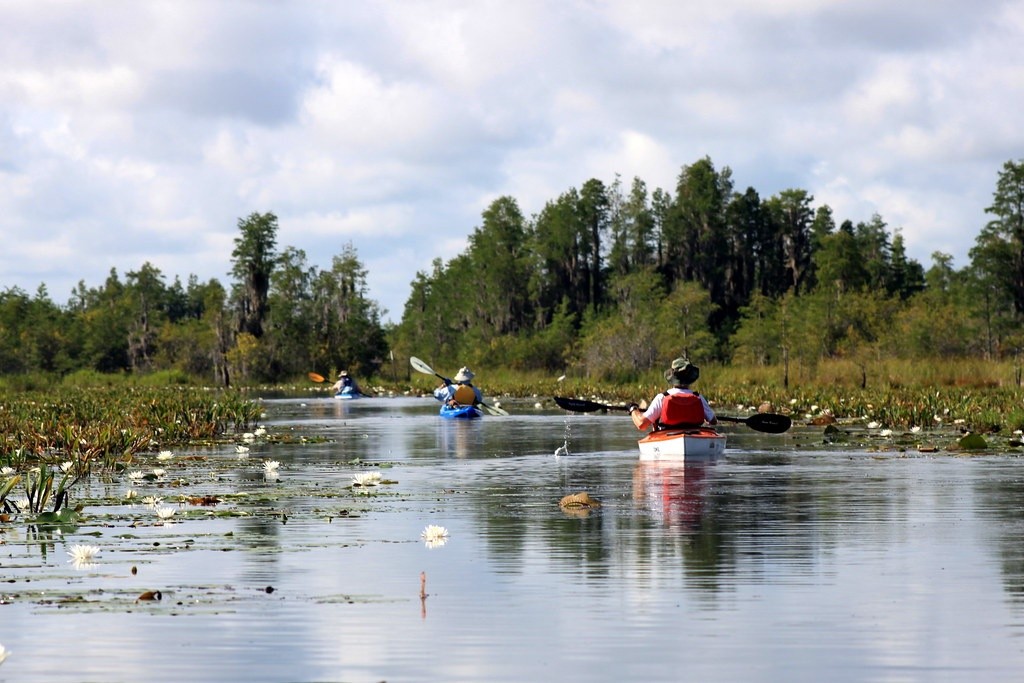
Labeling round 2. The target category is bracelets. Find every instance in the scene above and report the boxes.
[628,406,637,416]
[441,384,445,388]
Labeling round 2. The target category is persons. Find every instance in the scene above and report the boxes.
[624,357,717,431]
[332,371,359,395]
[433,366,483,408]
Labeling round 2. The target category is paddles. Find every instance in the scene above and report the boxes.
[553,396,791,434]
[308,372,372,397]
[409,356,509,416]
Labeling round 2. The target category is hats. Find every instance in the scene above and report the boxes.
[337,370,349,377]
[454,366,475,381]
[664,356,700,387]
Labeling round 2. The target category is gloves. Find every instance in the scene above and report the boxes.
[625,402,639,414]
[444,378,451,386]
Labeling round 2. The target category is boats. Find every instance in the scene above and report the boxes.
[440,403,486,421]
[335,390,360,400]
[638,425,728,462]
